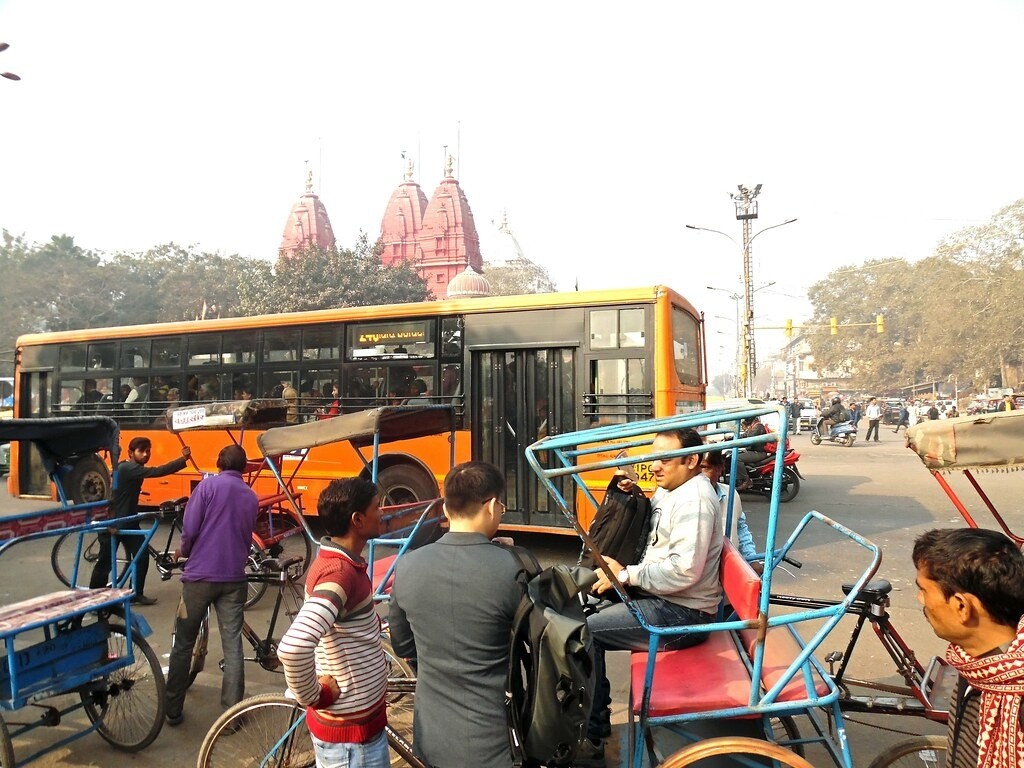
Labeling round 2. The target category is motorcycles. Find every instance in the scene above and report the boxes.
[715,436,806,503]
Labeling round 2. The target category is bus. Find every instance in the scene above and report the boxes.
[0,375,36,477]
[9,285,710,539]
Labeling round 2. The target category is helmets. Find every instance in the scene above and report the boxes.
[831,397,841,406]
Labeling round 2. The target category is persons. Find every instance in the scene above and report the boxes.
[820,398,825,409]
[928,404,939,420]
[87,437,191,603]
[566,428,723,765]
[387,460,528,768]
[165,445,260,734]
[69,380,103,416]
[826,399,831,407]
[999,395,1016,412]
[428,342,461,405]
[947,406,960,419]
[906,401,919,427]
[725,416,768,489]
[779,397,790,413]
[819,397,844,436]
[277,477,391,768]
[865,397,880,442]
[700,451,764,577]
[121,377,431,423]
[913,527,1024,768]
[848,402,860,426]
[892,403,908,433]
[790,397,805,435]
[940,402,947,419]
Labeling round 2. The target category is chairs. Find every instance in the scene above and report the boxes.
[95,394,113,416]
[137,388,168,423]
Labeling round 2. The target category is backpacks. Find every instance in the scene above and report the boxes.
[835,404,850,422]
[750,422,777,453]
[577,475,651,600]
[904,409,909,420]
[492,542,596,768]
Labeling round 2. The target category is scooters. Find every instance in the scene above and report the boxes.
[809,408,859,448]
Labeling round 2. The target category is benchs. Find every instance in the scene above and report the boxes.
[0,501,136,640]
[242,455,303,507]
[629,537,762,715]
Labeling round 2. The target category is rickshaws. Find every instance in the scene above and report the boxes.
[49,395,313,611]
[0,416,172,768]
[169,407,463,707]
[758,407,1024,768]
[191,405,888,768]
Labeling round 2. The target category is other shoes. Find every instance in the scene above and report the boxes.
[164,712,184,725]
[87,610,107,616]
[588,708,611,737]
[572,738,606,768]
[736,480,753,490]
[892,430,897,433]
[129,595,157,606]
[219,712,246,736]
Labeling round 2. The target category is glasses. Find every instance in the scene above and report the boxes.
[483,497,506,514]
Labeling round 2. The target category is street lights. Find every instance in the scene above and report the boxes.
[684,217,799,399]
[706,281,776,398]
[947,373,958,411]
[926,375,936,402]
[907,377,916,403]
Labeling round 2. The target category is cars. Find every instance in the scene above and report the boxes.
[844,389,1024,426]
[788,397,820,432]
